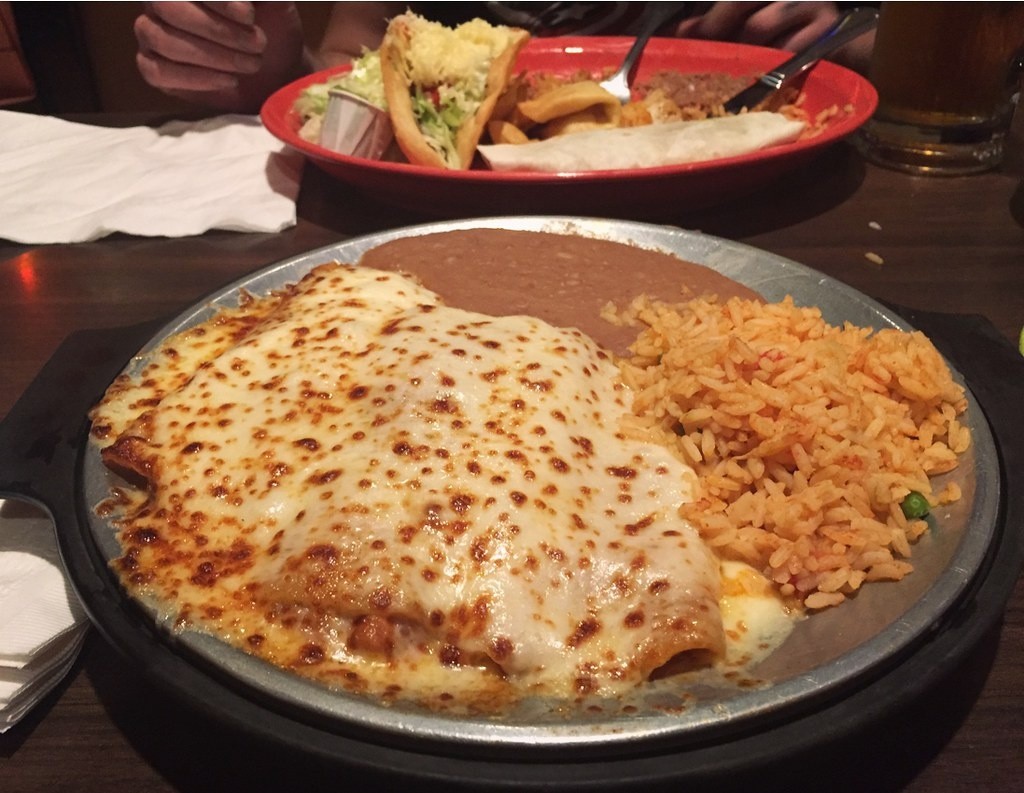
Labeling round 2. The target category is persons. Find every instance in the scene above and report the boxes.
[0,1,1024,167]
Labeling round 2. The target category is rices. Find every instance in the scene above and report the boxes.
[601,288,970,609]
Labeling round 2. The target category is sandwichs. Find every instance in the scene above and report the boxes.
[379,8,532,171]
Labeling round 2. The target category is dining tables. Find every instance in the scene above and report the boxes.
[3,111,1024,793]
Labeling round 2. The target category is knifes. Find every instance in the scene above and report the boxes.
[722,2,882,114]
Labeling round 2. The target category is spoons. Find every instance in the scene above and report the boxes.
[595,1,691,102]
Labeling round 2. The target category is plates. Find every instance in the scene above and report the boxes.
[58,209,1024,793]
[260,36,881,204]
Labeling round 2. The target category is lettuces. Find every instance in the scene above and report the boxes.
[296,44,386,144]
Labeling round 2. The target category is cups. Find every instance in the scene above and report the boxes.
[856,2,1024,177]
